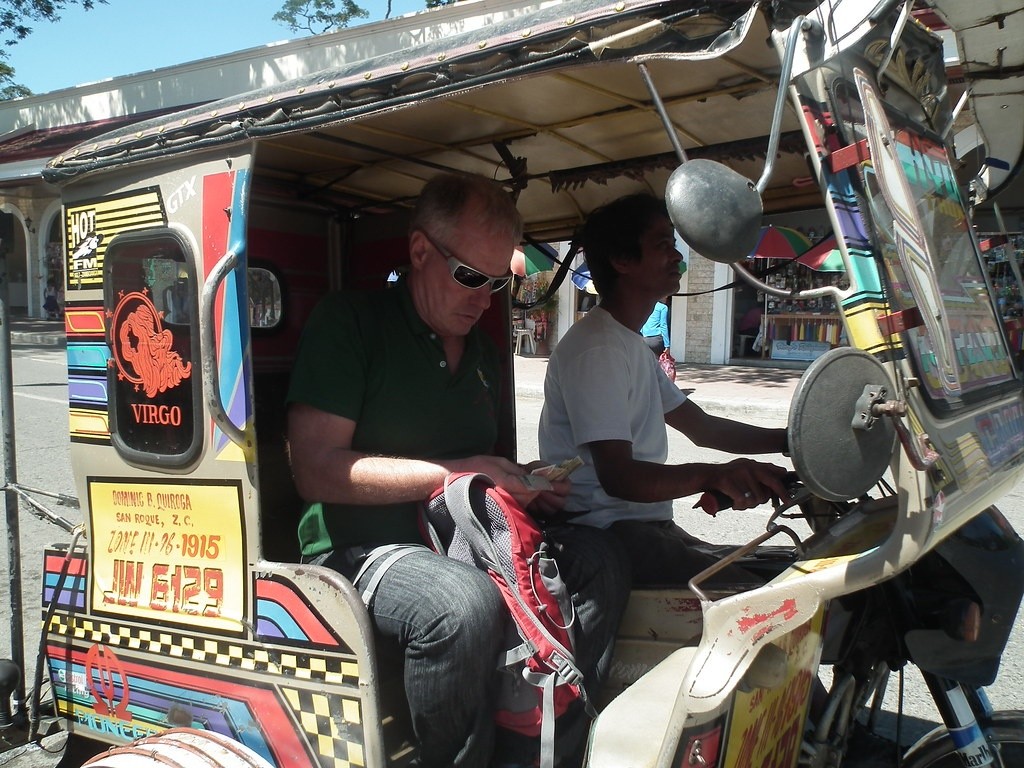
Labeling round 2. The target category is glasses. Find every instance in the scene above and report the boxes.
[414,224,514,293]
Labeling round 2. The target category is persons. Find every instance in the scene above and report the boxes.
[540,193,912,767]
[739,303,769,359]
[284,172,632,768]
[43,279,60,320]
[641,295,670,361]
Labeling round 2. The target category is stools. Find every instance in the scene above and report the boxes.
[732,334,759,358]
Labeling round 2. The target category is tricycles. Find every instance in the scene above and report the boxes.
[39,0,1024,768]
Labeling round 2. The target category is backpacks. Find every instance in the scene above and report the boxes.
[416,471,584,737]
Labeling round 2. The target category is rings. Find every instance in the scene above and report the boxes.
[745,491,751,498]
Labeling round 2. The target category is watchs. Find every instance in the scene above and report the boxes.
[782,427,791,457]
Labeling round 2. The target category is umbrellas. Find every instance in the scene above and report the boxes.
[744,224,815,339]
[510,243,558,277]
[572,259,686,296]
[795,233,847,272]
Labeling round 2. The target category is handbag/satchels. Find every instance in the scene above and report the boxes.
[659,353,676,384]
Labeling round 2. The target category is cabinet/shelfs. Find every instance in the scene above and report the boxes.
[766,231,1024,361]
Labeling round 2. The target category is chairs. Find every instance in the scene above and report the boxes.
[514,324,537,356]
